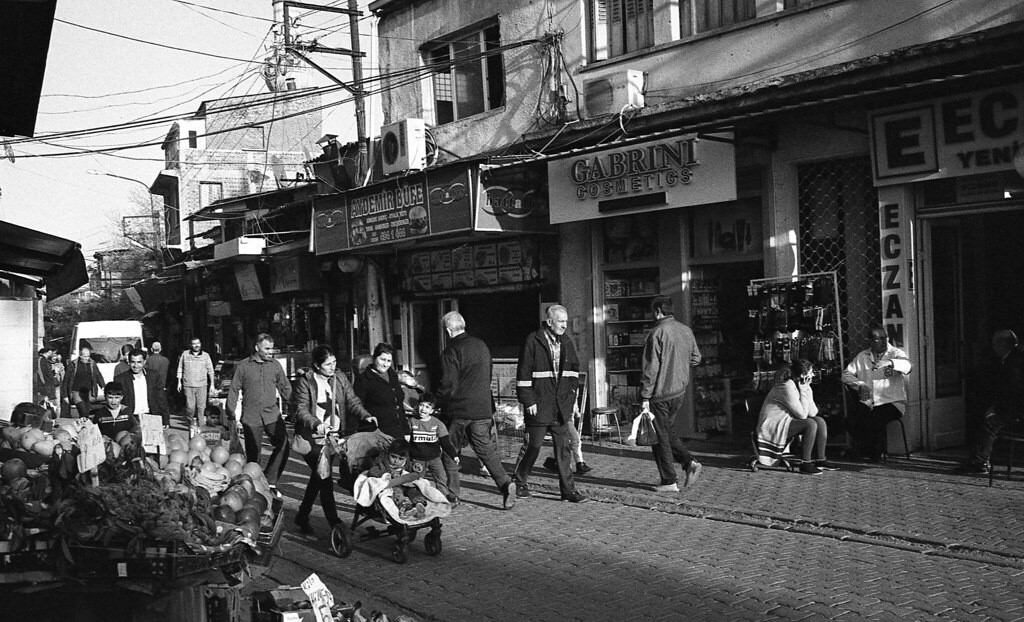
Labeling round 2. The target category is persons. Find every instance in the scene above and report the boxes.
[290,344,378,535]
[406,392,460,505]
[34,345,66,417]
[114,344,134,377]
[349,342,412,438]
[841,327,912,460]
[196,408,229,451]
[114,350,170,431]
[366,438,426,519]
[636,296,702,493]
[60,347,106,419]
[93,382,142,441]
[507,305,588,502]
[144,342,169,391]
[431,311,516,510]
[953,329,1024,476]
[176,337,216,439]
[565,395,594,475]
[226,335,294,499]
[755,359,841,476]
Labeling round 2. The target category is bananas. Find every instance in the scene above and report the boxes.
[185,461,229,494]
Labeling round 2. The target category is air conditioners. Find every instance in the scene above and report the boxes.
[584,70,644,119]
[383,117,427,177]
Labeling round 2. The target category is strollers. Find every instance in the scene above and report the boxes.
[325,419,451,564]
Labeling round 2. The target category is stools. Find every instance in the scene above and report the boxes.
[591,407,622,446]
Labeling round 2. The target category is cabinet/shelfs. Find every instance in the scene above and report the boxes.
[604,293,658,422]
[694,279,751,431]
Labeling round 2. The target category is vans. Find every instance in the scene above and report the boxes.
[68,321,148,404]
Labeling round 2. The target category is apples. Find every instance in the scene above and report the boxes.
[211,474,268,525]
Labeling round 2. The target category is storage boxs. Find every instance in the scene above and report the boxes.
[0,496,286,585]
[604,275,655,424]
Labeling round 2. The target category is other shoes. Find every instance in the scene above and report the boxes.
[502,481,516,510]
[414,501,427,519]
[575,461,593,477]
[269,485,283,498]
[514,481,532,499]
[654,482,680,492]
[446,494,459,504]
[954,463,990,476]
[684,461,701,488]
[560,490,588,503]
[799,458,823,475]
[816,460,840,470]
[399,505,417,520]
[870,452,885,465]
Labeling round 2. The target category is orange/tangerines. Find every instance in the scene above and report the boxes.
[160,433,230,470]
[223,453,273,510]
[115,430,139,450]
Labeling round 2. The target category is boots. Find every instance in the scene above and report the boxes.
[294,505,314,535]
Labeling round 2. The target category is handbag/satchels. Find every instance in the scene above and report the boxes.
[317,434,331,480]
[627,406,659,446]
[229,419,244,457]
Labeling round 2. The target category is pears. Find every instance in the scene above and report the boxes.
[0,425,78,459]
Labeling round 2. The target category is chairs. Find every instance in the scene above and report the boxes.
[845,385,910,460]
[981,418,1024,486]
[747,396,801,471]
